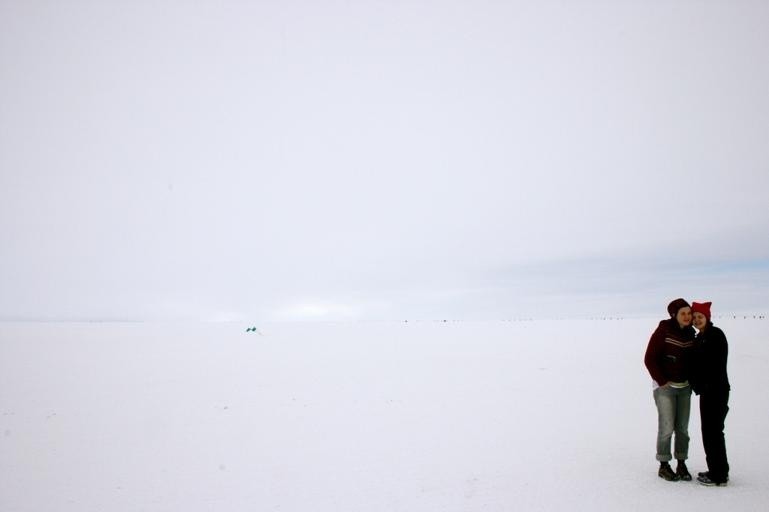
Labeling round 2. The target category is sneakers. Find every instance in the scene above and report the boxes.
[697,470,729,486]
[659,465,680,481]
[677,465,692,481]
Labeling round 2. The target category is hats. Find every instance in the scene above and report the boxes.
[668,299,690,317]
[691,302,712,322]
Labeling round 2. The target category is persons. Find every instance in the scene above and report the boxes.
[644,298,730,487]
